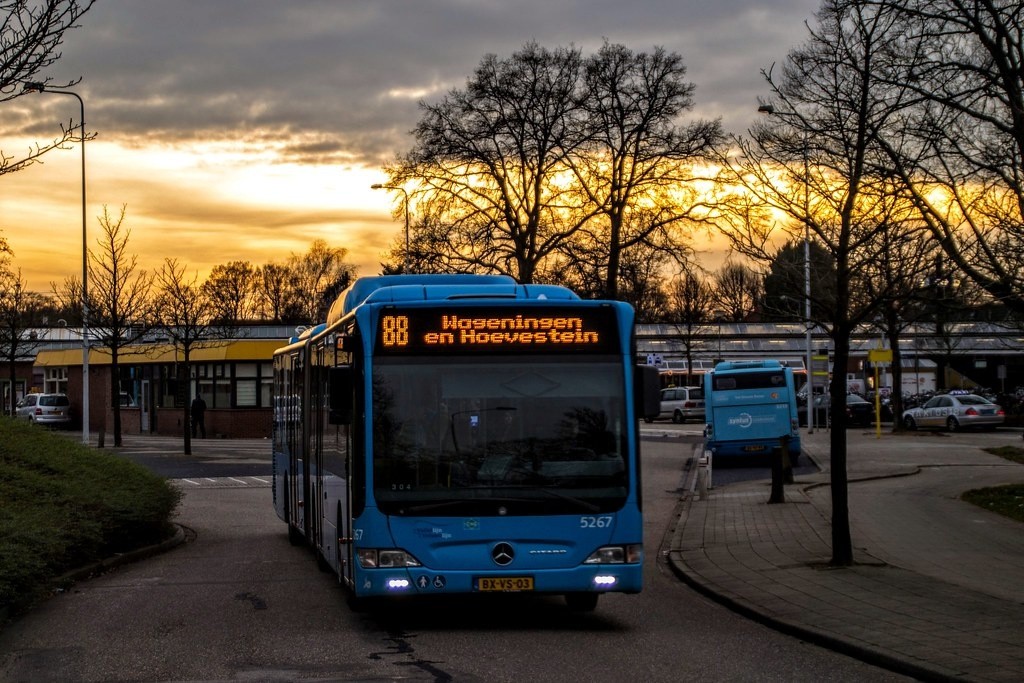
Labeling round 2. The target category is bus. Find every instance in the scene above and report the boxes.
[703,360,802,473]
[271,276,662,622]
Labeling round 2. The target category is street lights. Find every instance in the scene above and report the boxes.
[23,81,90,446]
[370,185,409,275]
[759,104,815,437]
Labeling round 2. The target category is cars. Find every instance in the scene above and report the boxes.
[902,392,1004,434]
[16,392,72,427]
[797,392,873,429]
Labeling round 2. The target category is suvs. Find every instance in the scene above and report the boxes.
[644,386,705,424]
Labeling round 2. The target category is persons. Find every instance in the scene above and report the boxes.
[190,392,207,439]
[487,410,517,454]
[544,415,597,461]
